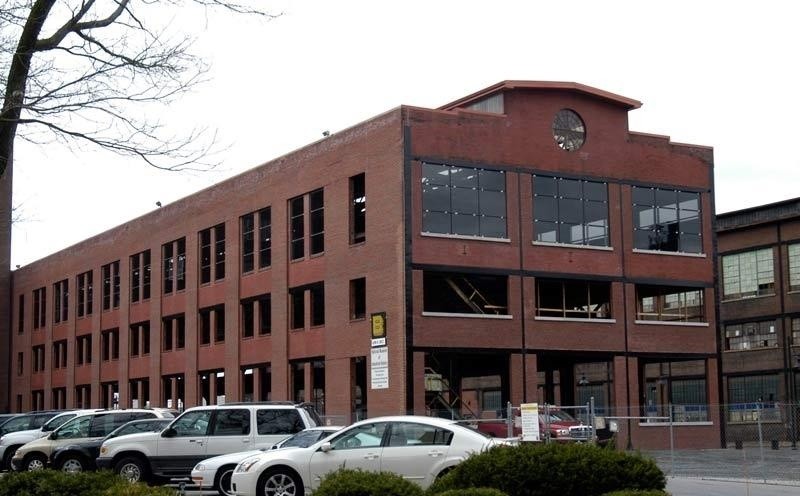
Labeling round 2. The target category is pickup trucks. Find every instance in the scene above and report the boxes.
[477,404,592,443]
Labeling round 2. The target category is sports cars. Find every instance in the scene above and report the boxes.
[188,423,424,496]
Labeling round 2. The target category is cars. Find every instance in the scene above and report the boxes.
[228,413,523,495]
